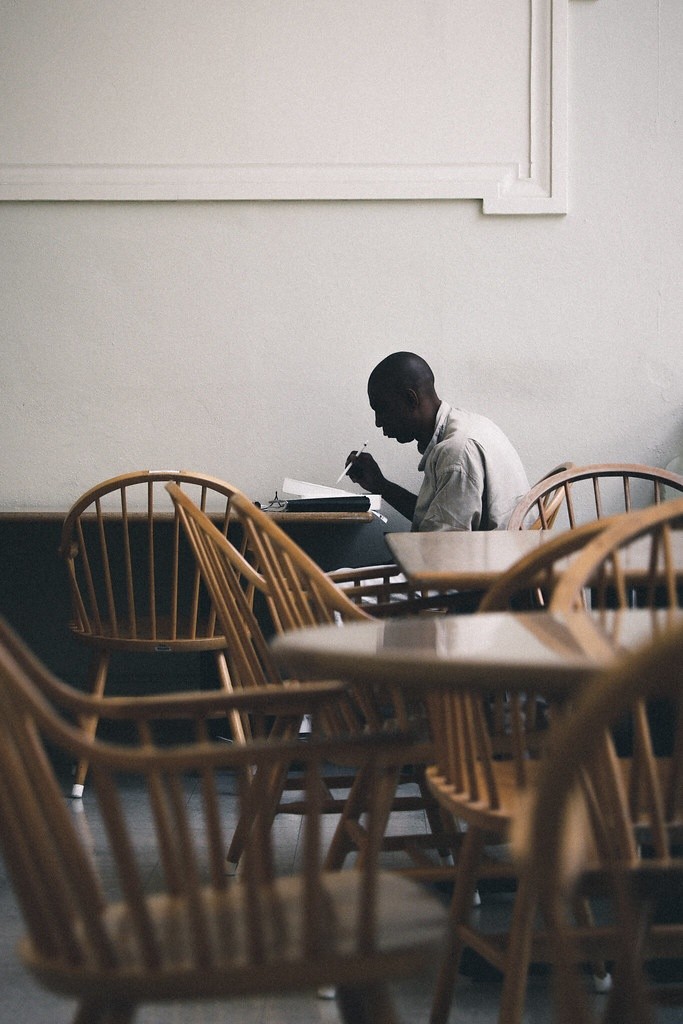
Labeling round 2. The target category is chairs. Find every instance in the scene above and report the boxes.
[0,462,683,1024]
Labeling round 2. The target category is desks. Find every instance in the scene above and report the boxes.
[0,506,376,788]
[268,609,683,1024]
[386,526,683,909]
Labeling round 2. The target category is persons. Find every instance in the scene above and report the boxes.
[297,351,541,734]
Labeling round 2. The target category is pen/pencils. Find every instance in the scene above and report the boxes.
[336,440,369,483]
